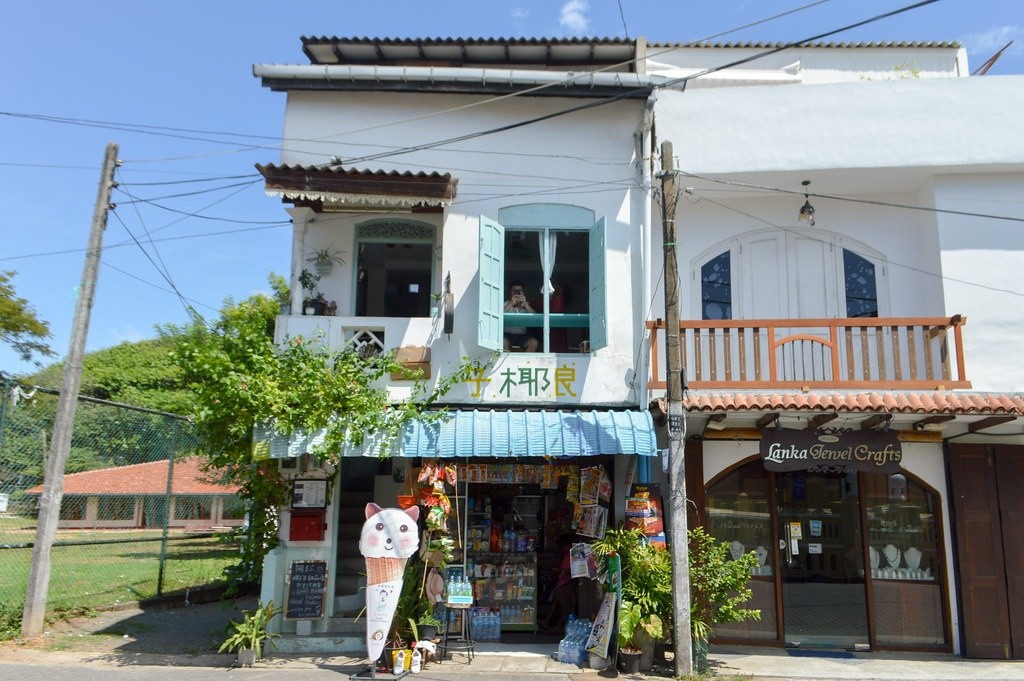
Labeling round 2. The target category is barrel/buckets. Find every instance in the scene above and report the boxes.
[392,649,411,671]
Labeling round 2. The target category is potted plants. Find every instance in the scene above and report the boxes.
[415,610,444,638]
[307,242,349,276]
[617,599,662,674]
[366,564,427,668]
[623,542,676,669]
[299,269,337,317]
[218,597,289,664]
[428,292,443,317]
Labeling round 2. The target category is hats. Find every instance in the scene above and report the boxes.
[425,567,443,606]
[419,529,446,562]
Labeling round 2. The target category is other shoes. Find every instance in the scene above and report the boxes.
[547,626,562,633]
[538,620,548,628]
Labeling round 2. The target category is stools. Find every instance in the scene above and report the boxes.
[438,602,478,664]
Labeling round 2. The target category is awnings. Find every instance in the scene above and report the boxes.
[253,408,659,461]
[254,163,457,207]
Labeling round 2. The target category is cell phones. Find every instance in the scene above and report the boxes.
[514,290,521,298]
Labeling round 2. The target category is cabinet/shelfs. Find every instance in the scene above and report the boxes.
[468,495,542,533]
[706,508,772,549]
[867,504,937,552]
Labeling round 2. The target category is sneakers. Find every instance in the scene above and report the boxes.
[393,650,405,674]
[411,650,421,674]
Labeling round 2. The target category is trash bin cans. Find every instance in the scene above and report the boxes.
[670,625,708,673]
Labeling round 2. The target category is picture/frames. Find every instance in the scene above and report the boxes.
[291,479,329,509]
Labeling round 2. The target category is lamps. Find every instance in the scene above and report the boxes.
[798,181,817,227]
[774,417,783,430]
[877,419,894,432]
[912,423,926,433]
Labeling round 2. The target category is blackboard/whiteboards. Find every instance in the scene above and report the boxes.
[283,559,327,621]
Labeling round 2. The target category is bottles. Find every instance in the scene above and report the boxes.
[442,568,472,600]
[467,557,482,576]
[557,633,589,664]
[472,612,501,640]
[433,603,462,633]
[470,604,533,623]
[492,524,534,552]
[566,614,591,635]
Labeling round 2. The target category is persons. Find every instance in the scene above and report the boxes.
[549,277,568,353]
[536,534,578,635]
[503,280,540,352]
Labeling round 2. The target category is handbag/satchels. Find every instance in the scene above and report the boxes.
[398,464,418,509]
